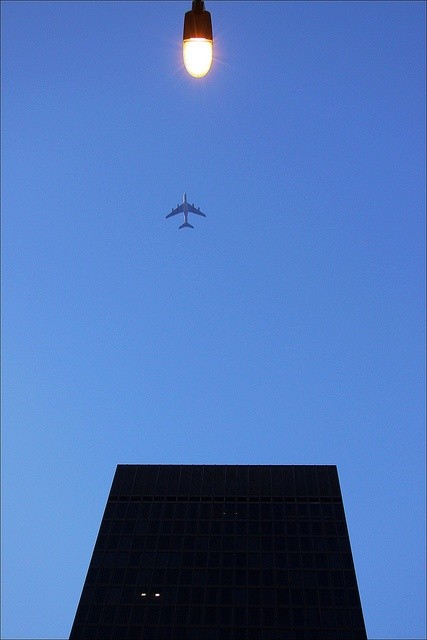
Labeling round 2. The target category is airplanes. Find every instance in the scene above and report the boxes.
[166,194,206,230]
